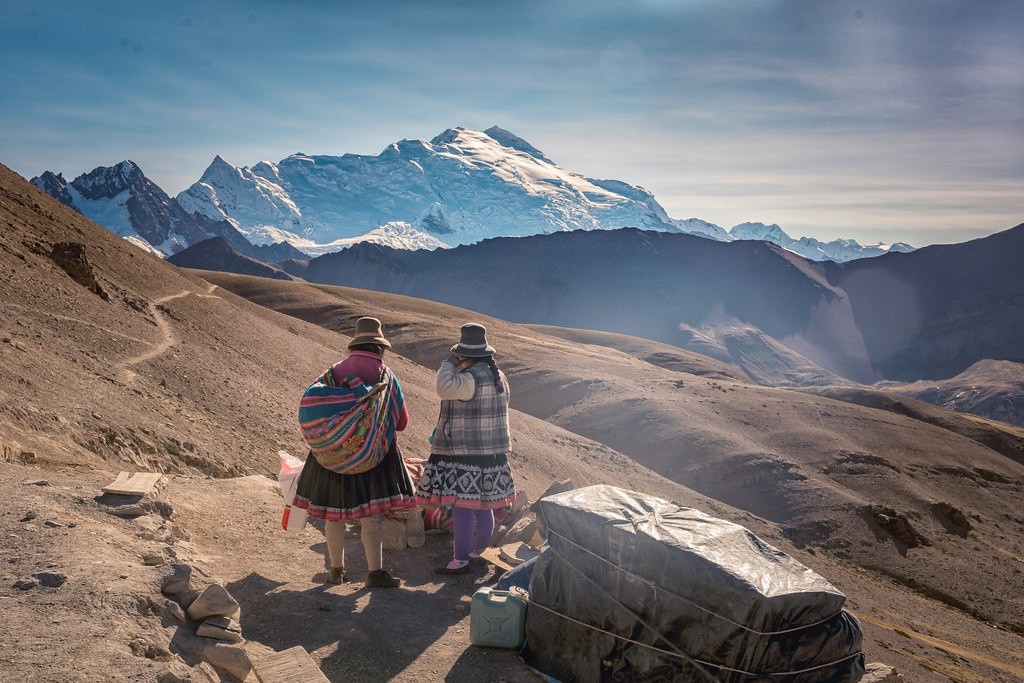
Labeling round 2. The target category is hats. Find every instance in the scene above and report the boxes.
[450,323,496,357]
[347,317,391,350]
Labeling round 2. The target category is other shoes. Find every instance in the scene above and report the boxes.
[469,551,489,567]
[435,563,471,575]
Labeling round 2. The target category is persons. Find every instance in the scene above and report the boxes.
[410,323,515,578]
[294,318,415,587]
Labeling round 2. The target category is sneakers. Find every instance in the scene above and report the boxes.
[365,570,401,588]
[328,569,346,585]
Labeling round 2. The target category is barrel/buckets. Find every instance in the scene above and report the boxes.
[470,585,528,650]
